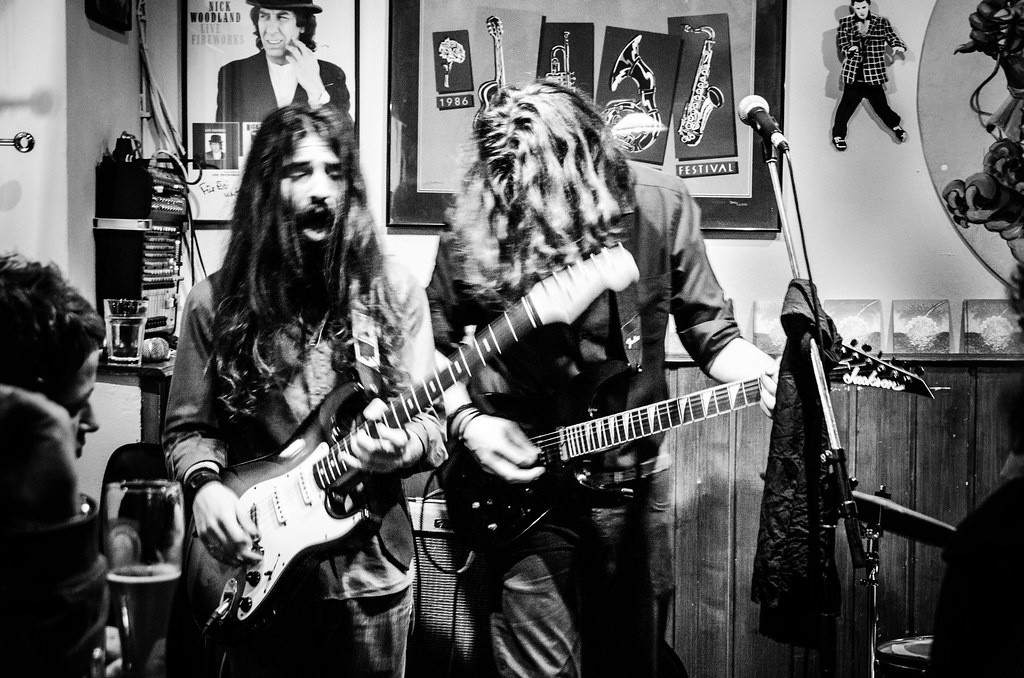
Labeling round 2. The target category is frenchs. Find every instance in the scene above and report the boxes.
[598,34,662,153]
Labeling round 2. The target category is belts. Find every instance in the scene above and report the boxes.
[558,449,672,489]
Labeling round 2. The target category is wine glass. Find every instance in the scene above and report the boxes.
[106,477,184,678]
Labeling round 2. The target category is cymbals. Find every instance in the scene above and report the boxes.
[852,490,956,547]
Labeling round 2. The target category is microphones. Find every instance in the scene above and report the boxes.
[738,95,789,152]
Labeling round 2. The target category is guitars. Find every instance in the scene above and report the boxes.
[181,242,639,642]
[444,333,936,555]
[472,16,506,131]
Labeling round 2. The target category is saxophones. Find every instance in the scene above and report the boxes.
[678,24,725,147]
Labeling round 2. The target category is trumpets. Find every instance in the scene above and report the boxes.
[545,31,576,90]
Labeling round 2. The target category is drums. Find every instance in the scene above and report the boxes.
[874,634,933,678]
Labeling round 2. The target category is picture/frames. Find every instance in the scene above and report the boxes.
[169,0,364,241]
[384,0,792,238]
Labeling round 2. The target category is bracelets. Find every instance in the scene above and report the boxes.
[447,403,483,443]
[186,472,223,515]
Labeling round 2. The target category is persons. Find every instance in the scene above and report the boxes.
[832,0,907,150]
[927,378,1024,678]
[0,252,108,461]
[207,134,225,161]
[164,103,449,678]
[424,77,781,678]
[215,0,351,165]
